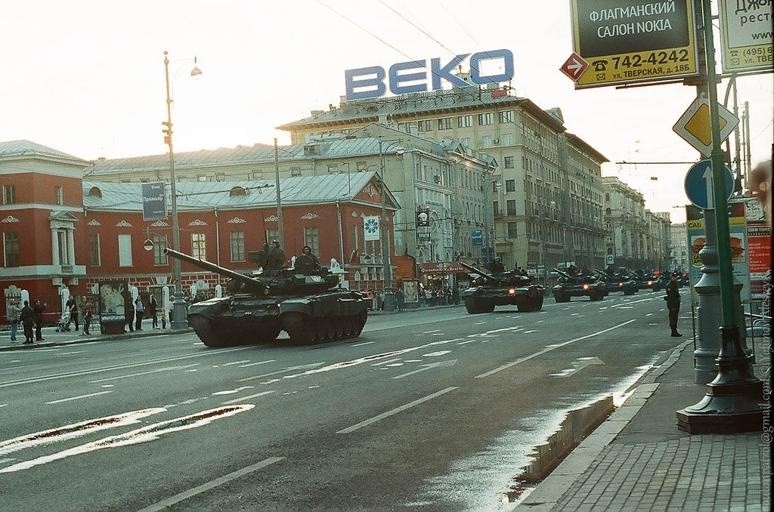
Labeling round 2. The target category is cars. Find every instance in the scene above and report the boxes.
[527,264,548,276]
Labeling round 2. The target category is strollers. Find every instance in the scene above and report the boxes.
[55,312,71,333]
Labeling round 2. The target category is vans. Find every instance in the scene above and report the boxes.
[556,260,577,269]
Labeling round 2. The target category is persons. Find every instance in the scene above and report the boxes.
[666,271,683,337]
[19,300,34,344]
[273,239,284,265]
[6,299,21,342]
[303,246,321,271]
[65,295,79,331]
[33,299,47,341]
[124,286,225,331]
[80,295,91,336]
[362,282,461,311]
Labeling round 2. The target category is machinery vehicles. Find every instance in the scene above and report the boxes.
[159,241,368,348]
[460,259,543,312]
[548,264,690,303]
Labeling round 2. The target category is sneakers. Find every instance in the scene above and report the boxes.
[10,339,45,344]
[672,331,682,336]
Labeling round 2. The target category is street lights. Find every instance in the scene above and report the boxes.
[376,135,402,310]
[158,48,205,331]
[534,190,556,283]
